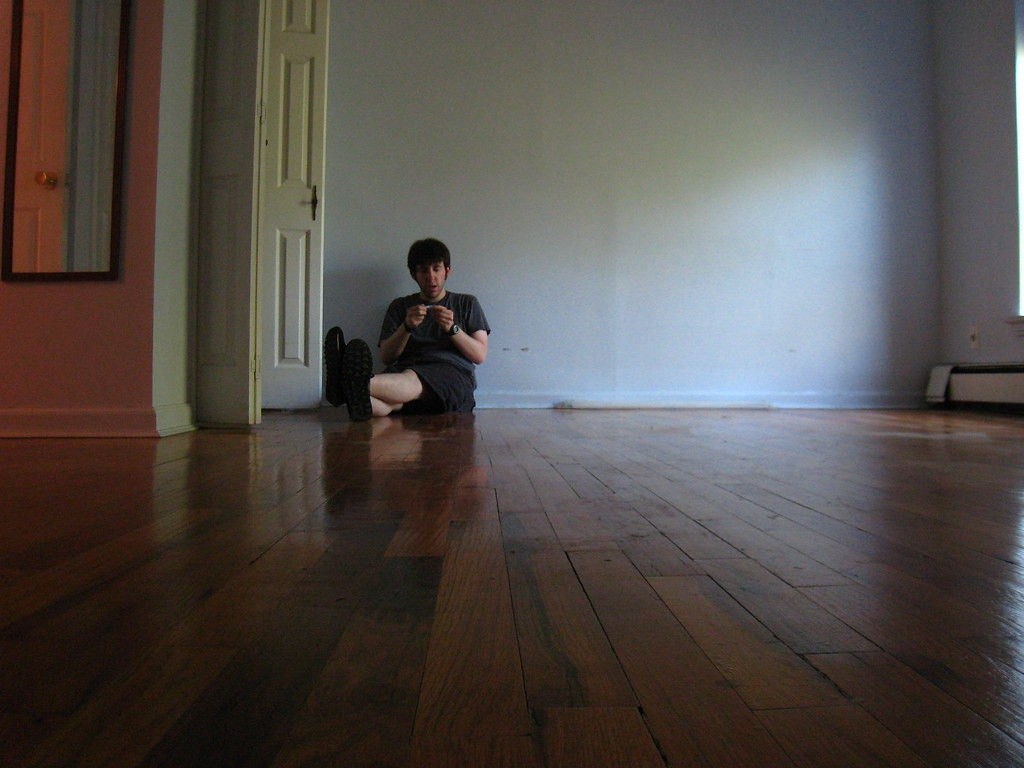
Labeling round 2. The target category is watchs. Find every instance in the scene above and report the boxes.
[445,322,459,336]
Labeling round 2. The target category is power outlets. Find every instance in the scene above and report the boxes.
[969,327,981,350]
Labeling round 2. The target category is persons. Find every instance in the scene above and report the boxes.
[325,238,491,422]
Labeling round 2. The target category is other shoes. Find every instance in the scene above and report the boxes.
[324,326,347,407]
[341,339,374,422]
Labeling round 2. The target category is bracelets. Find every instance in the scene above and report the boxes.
[403,321,415,334]
